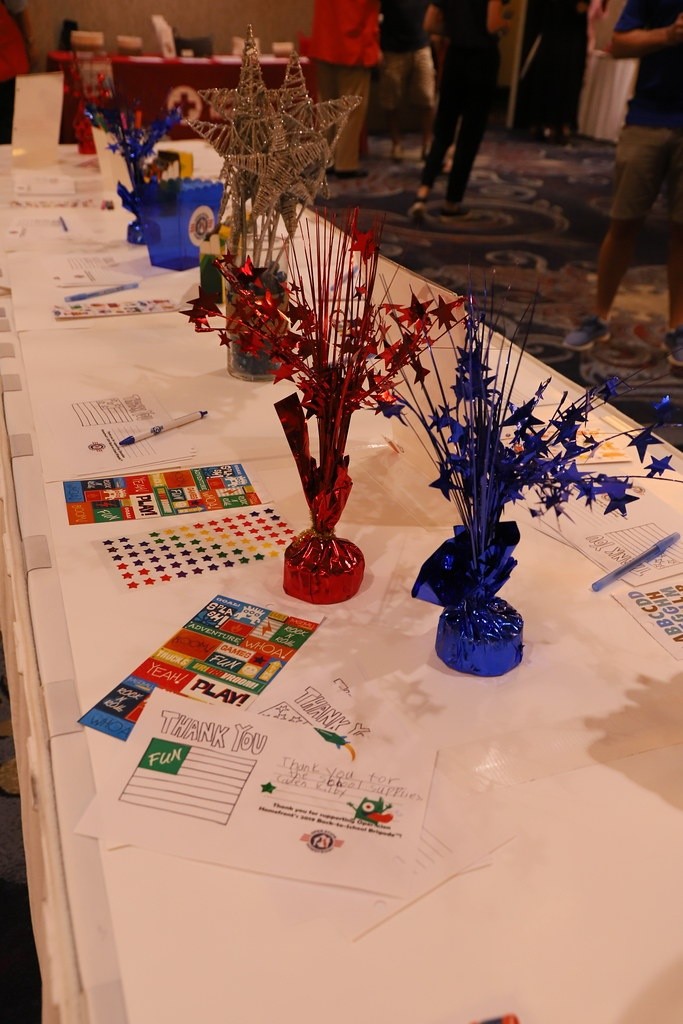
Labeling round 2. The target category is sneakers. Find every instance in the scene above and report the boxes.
[563,317,610,348]
[660,326,683,367]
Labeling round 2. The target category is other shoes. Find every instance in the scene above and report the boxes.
[422,154,430,162]
[326,166,368,179]
[408,200,437,225]
[390,156,404,164]
[440,207,472,223]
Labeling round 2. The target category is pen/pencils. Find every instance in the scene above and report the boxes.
[64,282,138,302]
[60,216,68,232]
[119,410,208,446]
[592,532,680,592]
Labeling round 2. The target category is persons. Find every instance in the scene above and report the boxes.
[381,0,438,164]
[560,0,683,368]
[0,0,36,143]
[308,0,384,178]
[407,0,510,234]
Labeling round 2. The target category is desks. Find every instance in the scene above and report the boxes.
[0,139,683,1024]
[111,54,318,165]
[47,50,164,107]
